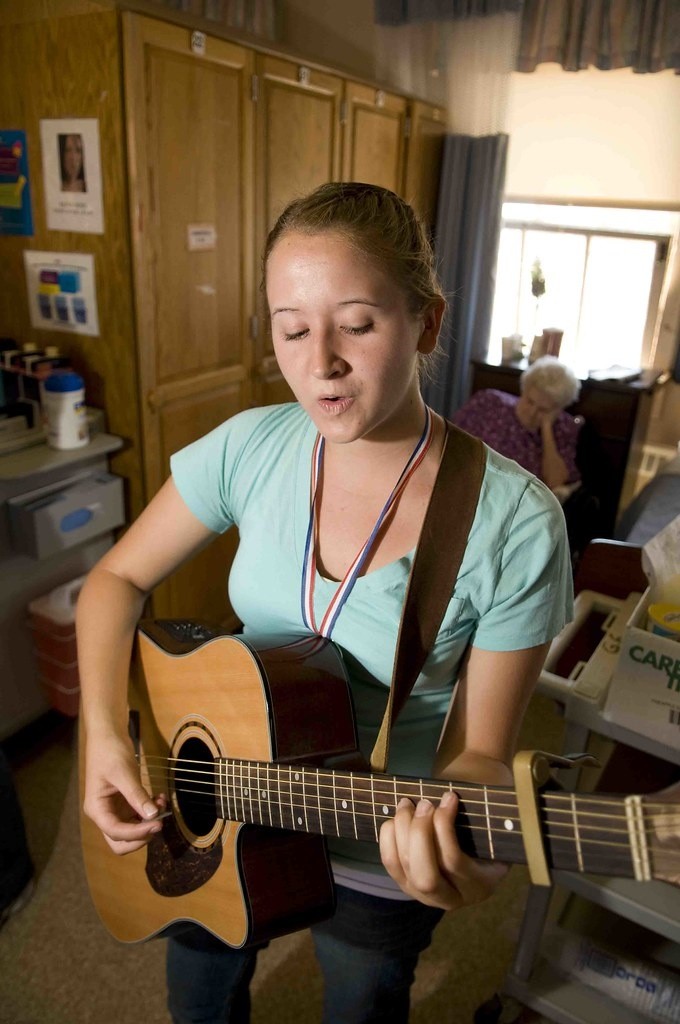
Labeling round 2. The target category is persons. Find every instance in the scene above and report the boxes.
[75,182,575,1024]
[450,355,579,492]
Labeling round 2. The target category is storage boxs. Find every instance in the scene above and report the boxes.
[603,585,680,751]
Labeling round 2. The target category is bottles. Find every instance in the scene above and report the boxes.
[43,369,89,449]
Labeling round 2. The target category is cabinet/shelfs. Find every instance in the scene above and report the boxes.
[472,589,680,1024]
[0,0,451,633]
[0,433,123,740]
[469,346,664,584]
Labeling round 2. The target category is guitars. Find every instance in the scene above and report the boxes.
[77,618,680,955]
